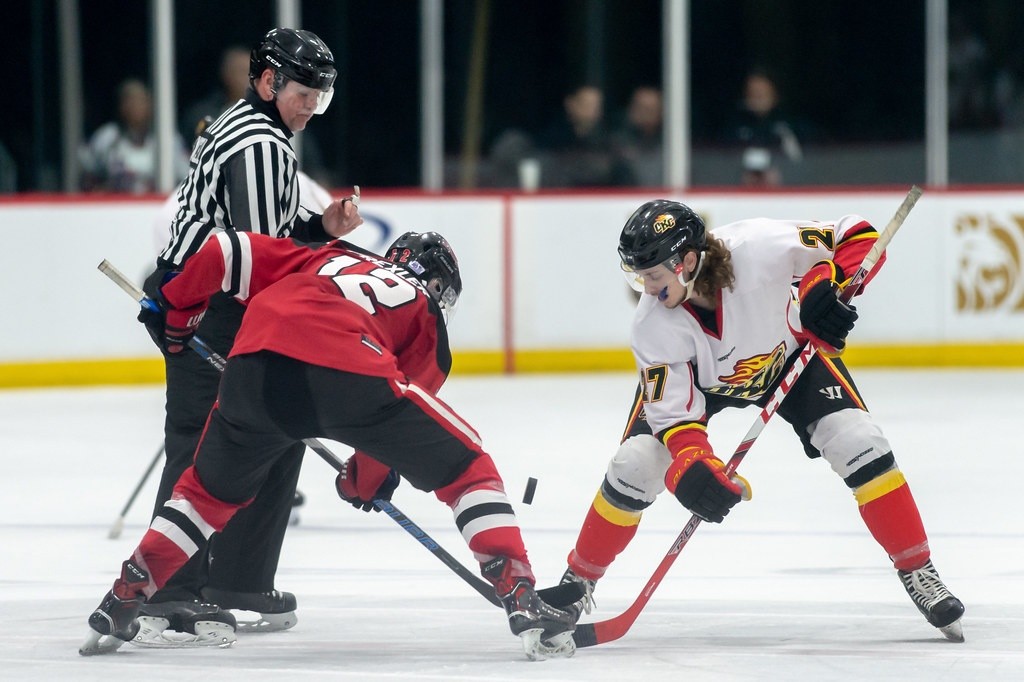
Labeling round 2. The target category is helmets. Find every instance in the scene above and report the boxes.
[248,27,337,101]
[384,230,463,320]
[618,198,704,269]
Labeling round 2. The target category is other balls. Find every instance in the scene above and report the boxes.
[522,477,538,505]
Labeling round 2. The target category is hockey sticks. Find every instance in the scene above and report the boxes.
[98,260,588,610]
[539,185,923,648]
[106,443,165,540]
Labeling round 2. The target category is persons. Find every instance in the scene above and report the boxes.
[539,76,621,186]
[720,71,807,189]
[522,196,966,663]
[90,79,192,190]
[606,89,664,187]
[77,229,581,663]
[193,46,255,120]
[155,111,336,526]
[135,28,364,647]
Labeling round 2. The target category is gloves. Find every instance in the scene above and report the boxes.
[663,449,752,524]
[798,259,859,352]
[135,270,209,355]
[335,444,401,512]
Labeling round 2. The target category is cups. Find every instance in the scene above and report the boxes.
[518,157,539,192]
[743,148,770,188]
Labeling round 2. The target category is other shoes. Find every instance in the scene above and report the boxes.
[286,491,304,525]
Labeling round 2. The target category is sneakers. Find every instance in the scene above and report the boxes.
[129,587,238,648]
[480,554,580,660]
[199,583,298,633]
[552,564,596,624]
[78,560,150,656]
[889,553,966,643]
